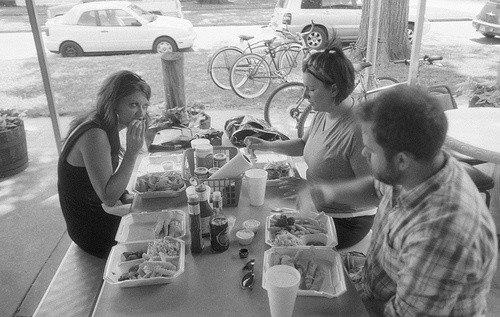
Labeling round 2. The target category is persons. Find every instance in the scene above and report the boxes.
[244,46,378,250]
[57,70,151,261]
[294,84,497,317]
[112,11,125,26]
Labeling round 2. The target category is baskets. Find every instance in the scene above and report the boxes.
[181,147,243,207]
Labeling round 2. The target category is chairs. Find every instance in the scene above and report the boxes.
[424,84,495,208]
[99,15,110,25]
[113,15,126,26]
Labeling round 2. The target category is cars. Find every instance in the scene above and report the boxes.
[269,0,417,51]
[471,0,500,39]
[44,1,198,58]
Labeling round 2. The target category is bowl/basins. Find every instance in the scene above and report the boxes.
[102,238,185,288]
[132,172,187,199]
[262,246,346,298]
[264,212,338,250]
[255,160,296,186]
[113,209,186,243]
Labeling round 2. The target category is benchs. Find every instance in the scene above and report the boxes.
[32,241,108,317]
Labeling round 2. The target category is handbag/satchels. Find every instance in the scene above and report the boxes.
[224,114,290,147]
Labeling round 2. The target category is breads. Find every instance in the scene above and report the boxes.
[136,173,184,192]
[274,251,326,292]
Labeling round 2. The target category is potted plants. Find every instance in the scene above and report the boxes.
[0,108,30,179]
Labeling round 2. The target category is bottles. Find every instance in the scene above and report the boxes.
[195,184,213,237]
[214,153,226,168]
[187,194,205,252]
[209,191,229,251]
[195,144,213,169]
[207,166,220,178]
[193,166,208,181]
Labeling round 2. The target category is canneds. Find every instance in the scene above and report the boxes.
[194,153,227,180]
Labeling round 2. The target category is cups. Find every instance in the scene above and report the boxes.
[190,138,211,147]
[186,184,211,203]
[236,230,256,244]
[162,160,172,170]
[243,219,261,234]
[265,264,301,317]
[244,168,267,205]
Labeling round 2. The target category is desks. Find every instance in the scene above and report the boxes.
[88,144,371,317]
[441,107,500,236]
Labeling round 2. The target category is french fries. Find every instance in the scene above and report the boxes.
[269,211,327,234]
[138,219,181,279]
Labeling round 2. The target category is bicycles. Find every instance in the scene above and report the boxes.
[207,20,311,90]
[263,37,443,141]
[229,17,318,101]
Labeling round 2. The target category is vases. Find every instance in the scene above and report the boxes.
[156,109,211,129]
